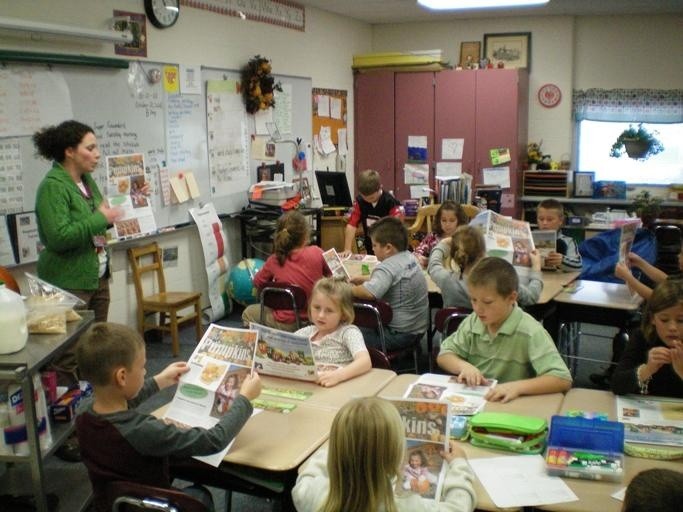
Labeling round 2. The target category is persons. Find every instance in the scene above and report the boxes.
[401,449,433,492]
[611,277,682,396]
[130,180,142,203]
[420,385,439,399]
[342,216,430,374]
[620,466,683,511]
[291,395,477,511]
[283,274,371,388]
[216,374,240,413]
[339,169,406,257]
[410,200,472,267]
[527,197,583,271]
[30,116,125,463]
[241,209,336,333]
[514,241,528,264]
[439,256,574,405]
[73,319,262,511]
[427,223,544,348]
[588,241,683,393]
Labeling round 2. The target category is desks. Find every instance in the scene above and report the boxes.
[321,206,364,252]
[297,374,564,510]
[554,279,646,381]
[532,386,683,511]
[139,348,399,507]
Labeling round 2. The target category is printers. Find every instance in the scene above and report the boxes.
[246,181,296,206]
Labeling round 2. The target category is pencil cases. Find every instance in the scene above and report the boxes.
[546,414,625,480]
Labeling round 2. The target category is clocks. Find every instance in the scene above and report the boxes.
[144,0,180,28]
[538,83,561,107]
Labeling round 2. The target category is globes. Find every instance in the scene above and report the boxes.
[226,257,265,306]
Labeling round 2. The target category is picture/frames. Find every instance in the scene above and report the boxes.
[292,177,312,201]
[483,31,531,71]
[573,170,595,198]
[460,40,480,67]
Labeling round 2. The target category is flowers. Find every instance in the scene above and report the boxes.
[525,140,551,164]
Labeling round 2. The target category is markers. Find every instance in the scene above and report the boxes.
[119,233,150,241]
[157,227,176,234]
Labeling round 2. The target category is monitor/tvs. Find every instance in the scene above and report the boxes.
[314,171,352,207]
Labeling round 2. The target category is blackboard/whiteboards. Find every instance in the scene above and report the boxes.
[0,50,315,271]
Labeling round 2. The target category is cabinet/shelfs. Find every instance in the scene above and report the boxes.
[355,73,435,213]
[241,208,323,263]
[522,169,568,196]
[0,309,101,509]
[520,195,682,237]
[436,70,529,218]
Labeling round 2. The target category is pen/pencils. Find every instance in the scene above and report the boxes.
[473,427,543,448]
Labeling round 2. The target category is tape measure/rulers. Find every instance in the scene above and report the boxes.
[261,387,312,400]
[250,398,297,412]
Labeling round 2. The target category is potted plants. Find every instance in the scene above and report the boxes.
[610,121,665,159]
[632,189,663,223]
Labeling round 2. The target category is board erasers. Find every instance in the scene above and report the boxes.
[218,214,231,220]
[176,223,190,229]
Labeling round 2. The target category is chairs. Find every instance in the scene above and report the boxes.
[103,482,211,511]
[128,241,202,356]
[407,203,483,240]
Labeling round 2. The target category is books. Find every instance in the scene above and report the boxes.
[531,230,555,271]
[435,171,473,206]
